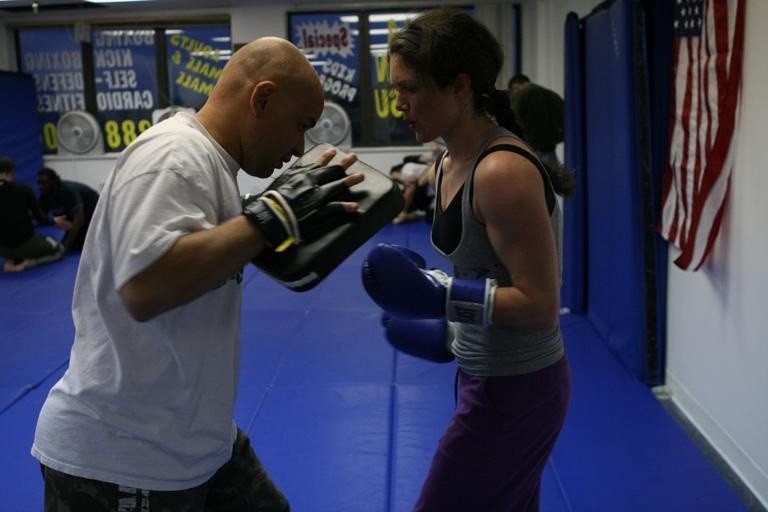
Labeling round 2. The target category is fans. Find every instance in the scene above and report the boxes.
[302,100,352,152]
[52,108,106,158]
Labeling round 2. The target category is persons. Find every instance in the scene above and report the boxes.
[390,76,562,225]
[31,37,365,512]
[0,156,64,272]
[362,14,569,510]
[36,168,100,253]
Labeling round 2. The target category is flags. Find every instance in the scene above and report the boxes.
[656,1,745,273]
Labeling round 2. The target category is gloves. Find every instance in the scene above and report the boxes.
[360,241,492,330]
[379,307,454,363]
